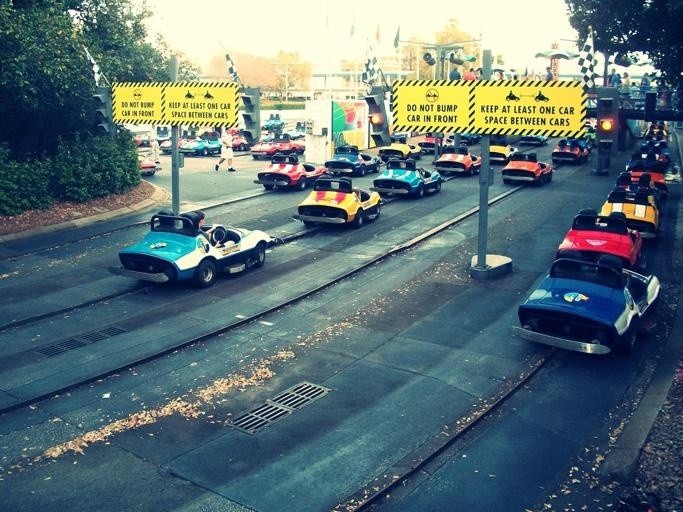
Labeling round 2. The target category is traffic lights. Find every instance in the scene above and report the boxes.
[364,82,391,146]
[239,86,261,141]
[594,86,620,156]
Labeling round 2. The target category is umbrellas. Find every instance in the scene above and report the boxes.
[534,49,580,72]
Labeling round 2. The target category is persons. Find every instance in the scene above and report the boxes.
[608,68,668,114]
[192,210,222,248]
[449,67,504,81]
[215,127,236,171]
[545,66,553,80]
